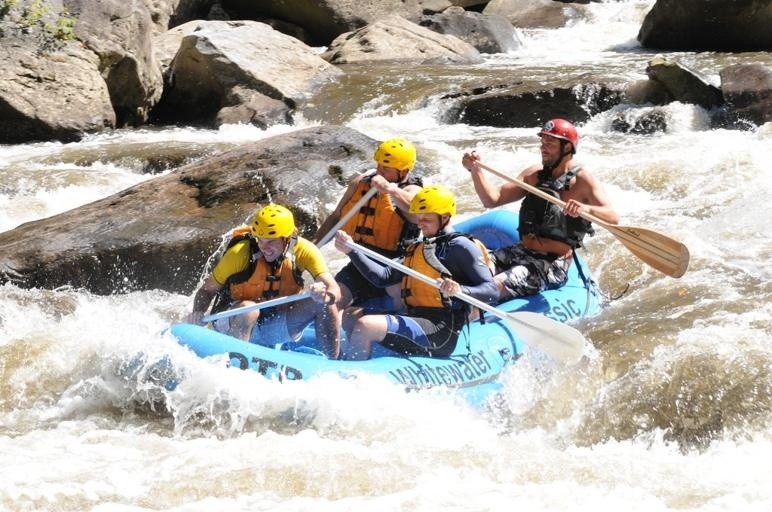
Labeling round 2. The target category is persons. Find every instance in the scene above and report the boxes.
[333,183,503,363]
[462,118,621,325]
[181,200,341,363]
[310,137,429,320]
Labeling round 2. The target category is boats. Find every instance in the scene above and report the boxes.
[157,206,604,407]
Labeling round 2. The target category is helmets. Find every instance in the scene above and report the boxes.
[409,185,457,216]
[374,139,416,171]
[250,205,298,239]
[537,118,577,153]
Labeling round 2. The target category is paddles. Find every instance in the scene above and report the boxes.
[335,231,585,364]
[464,149,689,278]
